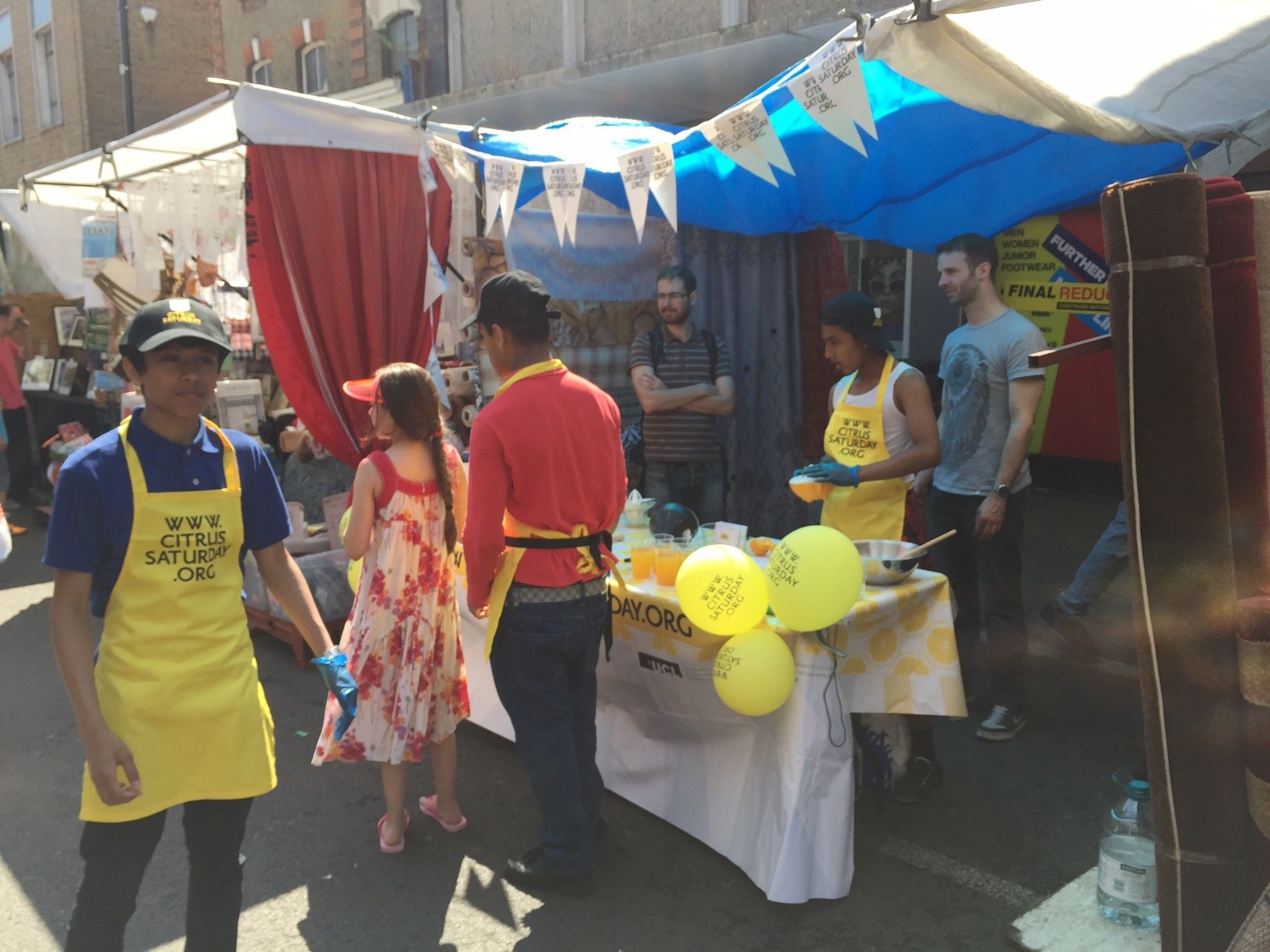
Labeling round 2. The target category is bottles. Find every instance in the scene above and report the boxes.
[1097,770,1159,930]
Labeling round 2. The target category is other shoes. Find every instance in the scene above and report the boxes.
[892,760,942,803]
[1038,592,1091,622]
[8,521,25,535]
[587,816,609,852]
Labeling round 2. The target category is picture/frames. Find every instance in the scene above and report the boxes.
[53,305,87,348]
[20,358,86,397]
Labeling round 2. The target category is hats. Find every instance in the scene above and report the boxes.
[117,297,232,367]
[823,290,896,355]
[342,373,384,406]
[459,268,561,330]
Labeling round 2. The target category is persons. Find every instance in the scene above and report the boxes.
[626,266,736,527]
[461,269,627,892]
[915,233,1047,740]
[1039,495,1130,661]
[0,302,35,563]
[42,298,358,952]
[310,362,469,855]
[793,291,942,800]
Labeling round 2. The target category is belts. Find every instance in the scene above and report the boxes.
[511,577,607,603]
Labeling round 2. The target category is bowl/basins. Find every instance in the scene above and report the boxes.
[854,539,927,586]
[789,475,834,503]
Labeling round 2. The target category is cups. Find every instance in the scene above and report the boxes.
[652,534,674,549]
[670,537,694,566]
[655,543,679,585]
[631,540,654,580]
[702,522,716,545]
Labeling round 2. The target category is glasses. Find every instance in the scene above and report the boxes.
[654,292,689,303]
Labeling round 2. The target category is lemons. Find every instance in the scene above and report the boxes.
[750,537,770,556]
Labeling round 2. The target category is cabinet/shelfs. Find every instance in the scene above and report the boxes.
[22,344,122,524]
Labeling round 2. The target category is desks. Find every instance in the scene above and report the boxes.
[452,513,968,903]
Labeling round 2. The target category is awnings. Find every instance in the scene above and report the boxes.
[0,0,1270,484]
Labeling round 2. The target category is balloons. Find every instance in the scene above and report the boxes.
[674,525,864,717]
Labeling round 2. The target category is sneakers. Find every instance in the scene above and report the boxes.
[975,700,1023,740]
[502,848,595,896]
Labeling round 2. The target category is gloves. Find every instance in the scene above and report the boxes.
[795,456,859,490]
[311,654,359,739]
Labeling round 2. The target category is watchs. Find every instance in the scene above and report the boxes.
[993,484,1010,497]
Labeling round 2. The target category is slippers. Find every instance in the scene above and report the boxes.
[420,795,467,831]
[377,810,410,853]
